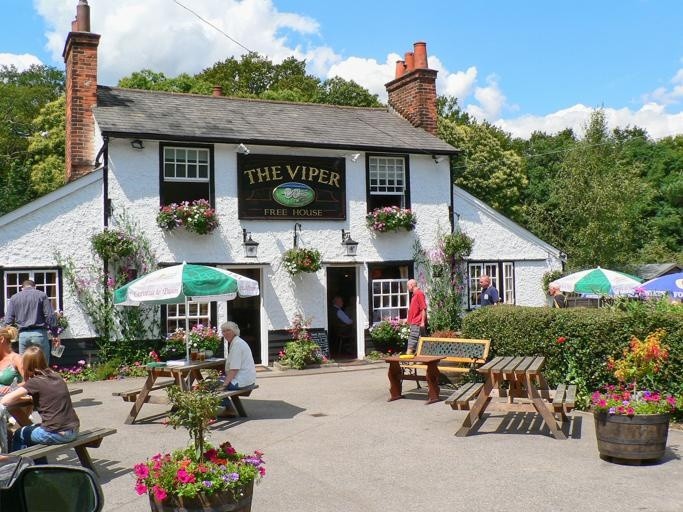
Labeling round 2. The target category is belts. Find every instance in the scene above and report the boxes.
[20,325,45,331]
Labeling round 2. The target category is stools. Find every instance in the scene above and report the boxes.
[333,333,354,353]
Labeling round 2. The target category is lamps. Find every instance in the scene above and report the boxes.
[243,233,260,258]
[352,154,361,163]
[341,233,359,256]
[131,141,144,149]
[238,144,250,156]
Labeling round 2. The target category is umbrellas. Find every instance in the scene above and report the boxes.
[114,260,259,389]
[551,266,641,308]
[639,273,683,302]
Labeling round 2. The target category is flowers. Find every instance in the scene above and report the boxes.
[156,198,219,235]
[48,309,69,337]
[366,205,417,233]
[590,327,677,417]
[89,229,137,282]
[281,246,322,278]
[427,239,445,264]
[134,369,266,503]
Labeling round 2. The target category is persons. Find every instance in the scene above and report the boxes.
[547,283,569,309]
[480,275,498,307]
[404,279,427,353]
[330,293,401,334]
[207,321,256,418]
[2,346,80,465]
[0,326,32,433]
[6,280,61,367]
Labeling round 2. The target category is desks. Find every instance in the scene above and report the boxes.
[383,355,446,405]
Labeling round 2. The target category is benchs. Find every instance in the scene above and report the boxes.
[400,337,490,389]
[0,388,117,479]
[545,384,577,417]
[112,380,174,397]
[195,385,259,417]
[445,382,485,411]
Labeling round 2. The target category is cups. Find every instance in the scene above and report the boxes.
[51,343,65,358]
[199,351,205,362]
[191,349,198,364]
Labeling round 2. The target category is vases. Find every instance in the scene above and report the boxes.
[149,474,254,512]
[592,412,670,460]
[433,265,442,278]
